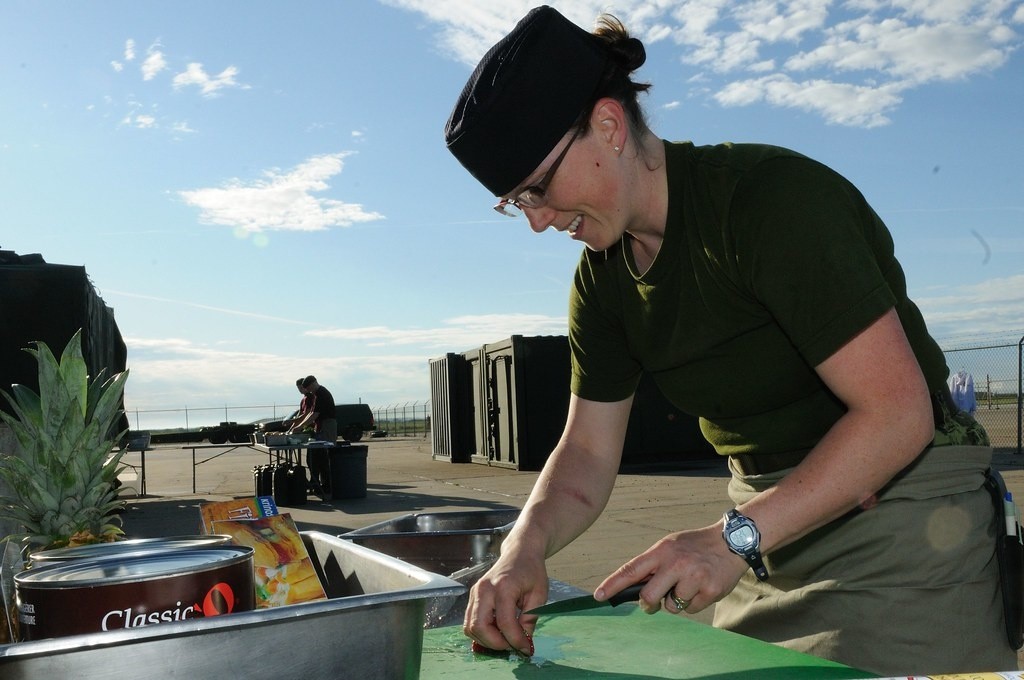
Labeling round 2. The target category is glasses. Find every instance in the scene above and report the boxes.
[492,128,586,218]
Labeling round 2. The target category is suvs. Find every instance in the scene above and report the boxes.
[259,404,376,443]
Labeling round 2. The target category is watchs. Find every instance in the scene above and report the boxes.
[721,508,771,583]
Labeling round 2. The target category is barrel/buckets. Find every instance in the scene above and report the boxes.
[27,534,233,571]
[11,545,259,642]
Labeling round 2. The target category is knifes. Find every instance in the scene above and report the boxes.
[524,581,654,618]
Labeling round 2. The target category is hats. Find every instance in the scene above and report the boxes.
[296,378,304,386]
[302,376,316,388]
[446,6,624,197]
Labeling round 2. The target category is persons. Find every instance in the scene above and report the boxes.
[444,4,1020,678]
[282,376,338,496]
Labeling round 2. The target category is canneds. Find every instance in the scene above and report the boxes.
[28,535,232,570]
[14,545,256,641]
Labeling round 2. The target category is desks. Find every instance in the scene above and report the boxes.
[255,441,335,506]
[419,573,884,679]
[109,447,154,497]
[182,443,303,493]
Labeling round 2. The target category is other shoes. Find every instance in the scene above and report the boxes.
[314,484,329,493]
[307,480,320,488]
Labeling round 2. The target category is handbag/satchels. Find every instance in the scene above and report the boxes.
[996,525,1024,650]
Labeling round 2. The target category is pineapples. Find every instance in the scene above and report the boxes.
[0,326,138,644]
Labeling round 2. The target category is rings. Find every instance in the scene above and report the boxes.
[670,589,694,610]
